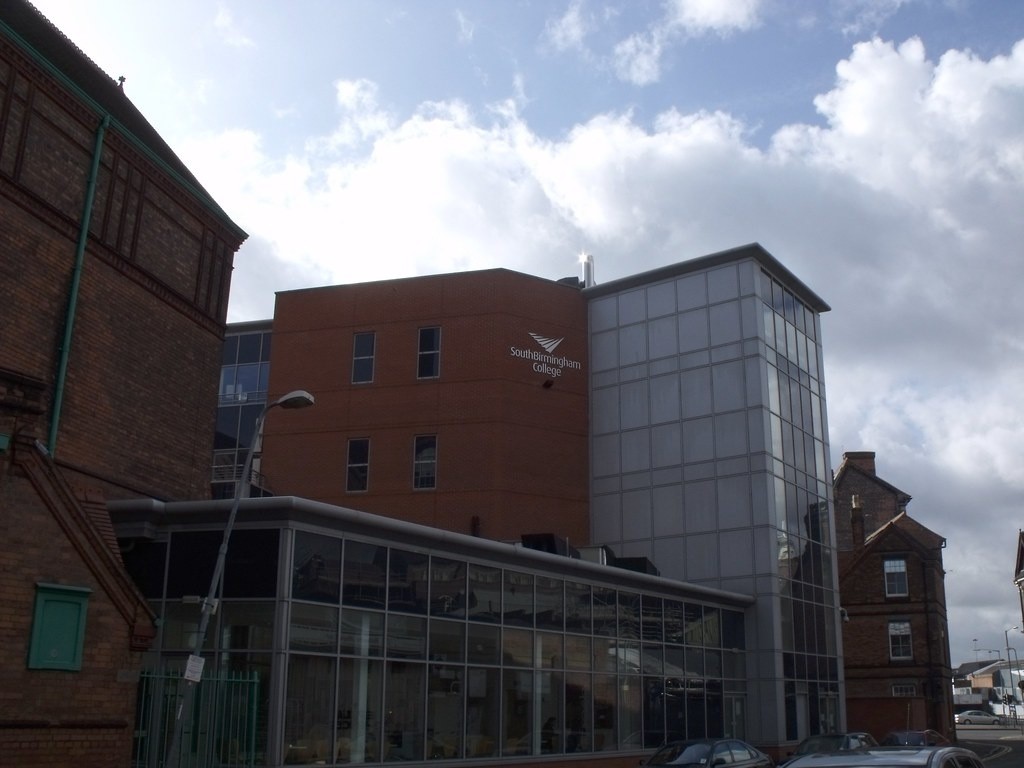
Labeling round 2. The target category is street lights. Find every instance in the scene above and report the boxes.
[1005,626,1019,725]
[992,649,1007,725]
[1005,647,1024,713]
[161,391,314,768]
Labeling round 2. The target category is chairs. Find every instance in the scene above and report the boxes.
[291,735,528,766]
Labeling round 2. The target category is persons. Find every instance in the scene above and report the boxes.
[540,716,559,752]
[566,725,583,753]
[426,728,436,759]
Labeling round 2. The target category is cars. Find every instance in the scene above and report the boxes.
[882,728,953,748]
[639,739,774,768]
[954,710,1001,725]
[772,748,985,768]
[775,732,879,767]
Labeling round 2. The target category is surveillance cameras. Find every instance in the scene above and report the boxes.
[843,616,849,624]
